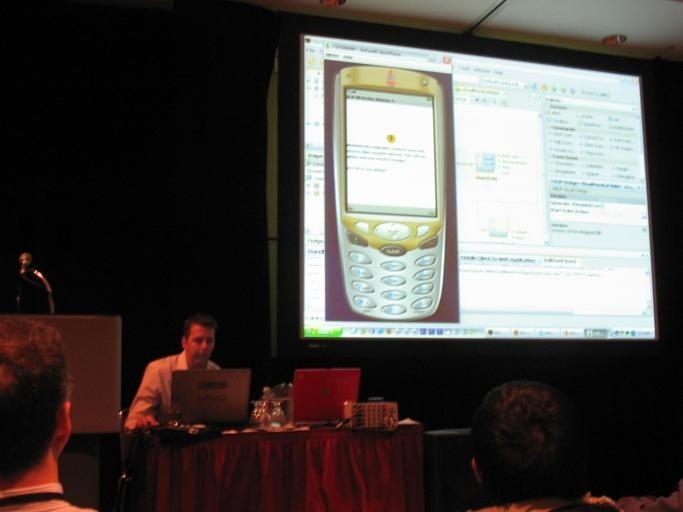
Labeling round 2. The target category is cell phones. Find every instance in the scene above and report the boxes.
[332,65,448,322]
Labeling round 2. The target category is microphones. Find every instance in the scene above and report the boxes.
[18,248,33,275]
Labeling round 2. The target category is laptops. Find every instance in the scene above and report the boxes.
[169,368,252,429]
[287,368,363,427]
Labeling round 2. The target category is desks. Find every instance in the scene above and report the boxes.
[143,424,421,511]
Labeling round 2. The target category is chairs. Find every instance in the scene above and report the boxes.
[114,406,140,511]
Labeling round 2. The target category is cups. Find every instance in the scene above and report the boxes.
[251,400,288,426]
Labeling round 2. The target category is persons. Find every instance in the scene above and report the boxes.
[0,318,99,512]
[124,314,222,431]
[16,253,54,314]
[467,381,573,512]
